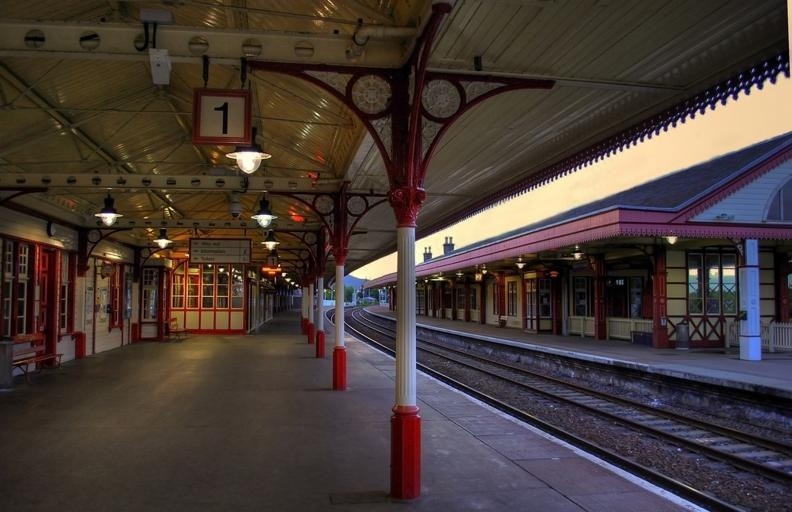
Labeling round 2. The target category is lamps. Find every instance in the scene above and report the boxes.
[382,235,679,290]
[277,262,302,289]
[226,127,271,175]
[206,263,241,280]
[250,192,278,229]
[94,191,124,226]
[261,228,280,251]
[153,228,173,249]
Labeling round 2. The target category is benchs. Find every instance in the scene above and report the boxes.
[167,316,188,342]
[10,332,64,385]
